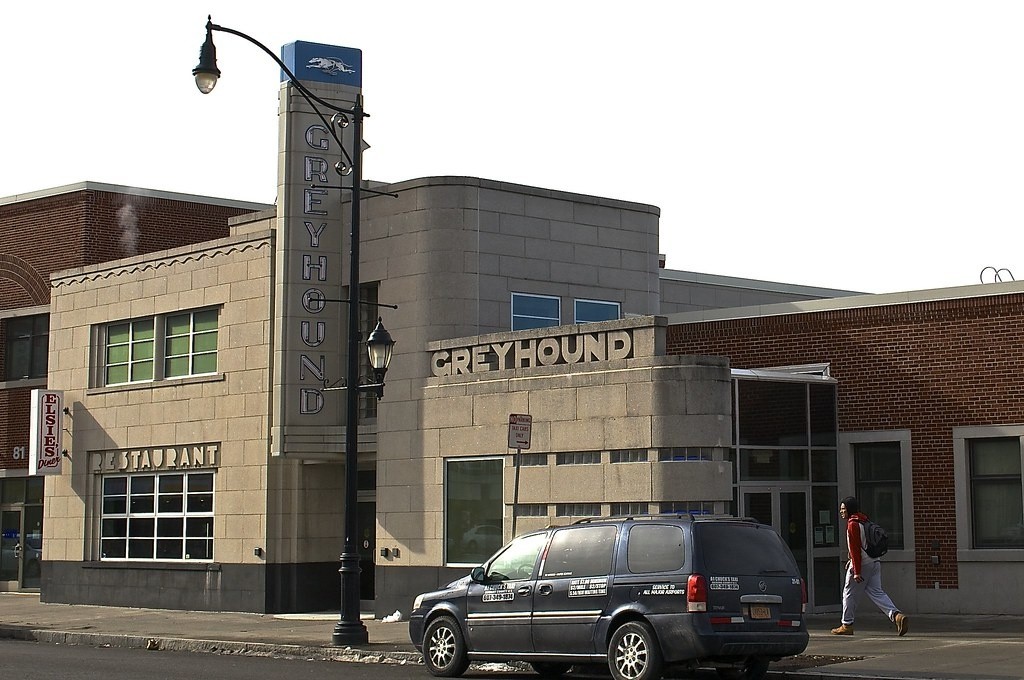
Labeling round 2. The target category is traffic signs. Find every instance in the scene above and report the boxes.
[508,414,532,451]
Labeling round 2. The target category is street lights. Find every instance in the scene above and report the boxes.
[195,21,395,647]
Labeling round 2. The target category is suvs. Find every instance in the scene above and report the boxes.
[407,510,808,680]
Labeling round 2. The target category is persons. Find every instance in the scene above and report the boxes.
[830,496,909,637]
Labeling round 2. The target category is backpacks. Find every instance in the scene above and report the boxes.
[846,517,888,558]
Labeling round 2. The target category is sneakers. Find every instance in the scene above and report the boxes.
[895,613,909,636]
[831,624,854,635]
